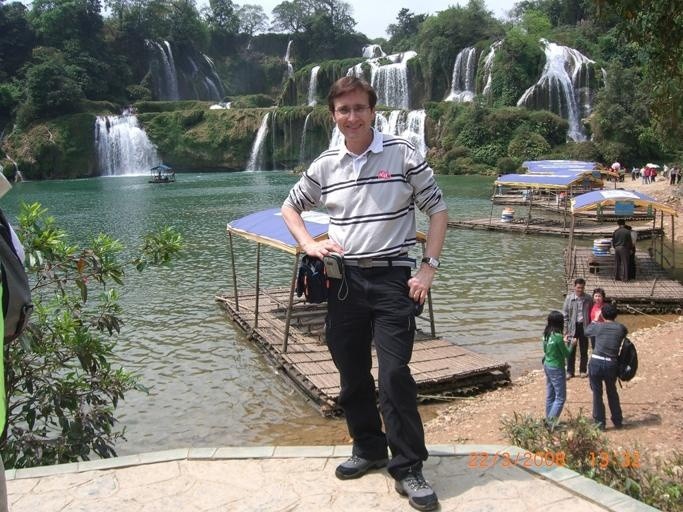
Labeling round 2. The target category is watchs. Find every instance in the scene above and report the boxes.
[420,256,441,271]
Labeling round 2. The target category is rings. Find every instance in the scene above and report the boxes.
[415,287,422,293]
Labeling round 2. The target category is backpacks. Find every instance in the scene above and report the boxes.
[615,324,638,381]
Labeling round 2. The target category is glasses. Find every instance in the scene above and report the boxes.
[334,105,370,113]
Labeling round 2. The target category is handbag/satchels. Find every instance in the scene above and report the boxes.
[323,251,347,281]
[295,250,330,305]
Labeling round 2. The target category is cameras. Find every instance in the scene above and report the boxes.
[567,336,575,340]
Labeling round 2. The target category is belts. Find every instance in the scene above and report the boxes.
[343,253,417,269]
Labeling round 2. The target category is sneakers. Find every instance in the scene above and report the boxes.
[394,459,438,511]
[335,447,390,480]
[565,371,588,380]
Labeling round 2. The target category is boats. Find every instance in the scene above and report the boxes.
[211,204,514,423]
[147,164,175,183]
[557,188,683,319]
[437,155,668,243]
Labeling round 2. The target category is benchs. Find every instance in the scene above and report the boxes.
[509,216,582,227]
[587,253,617,278]
[270,296,329,343]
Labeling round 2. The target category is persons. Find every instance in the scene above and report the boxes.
[560,277,593,380]
[540,310,577,418]
[601,162,682,187]
[622,223,637,279]
[583,302,627,431]
[611,217,630,282]
[279,76,449,511]
[0,171,29,511]
[590,288,608,354]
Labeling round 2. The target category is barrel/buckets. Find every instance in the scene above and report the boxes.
[594,239,611,256]
[501,209,515,223]
